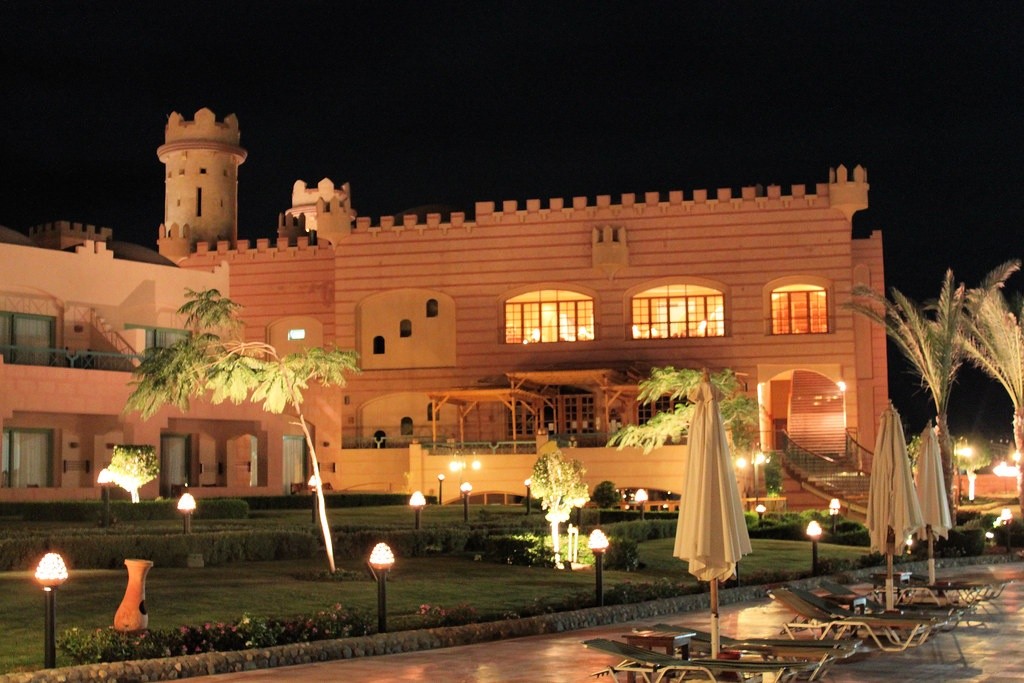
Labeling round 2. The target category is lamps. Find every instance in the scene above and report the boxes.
[308,474,322,492]
[755,505,766,515]
[588,528,610,556]
[409,490,426,511]
[437,474,445,481]
[33,551,69,587]
[97,468,112,488]
[806,520,823,540]
[176,492,197,515]
[635,488,648,505]
[460,482,473,495]
[369,542,395,572]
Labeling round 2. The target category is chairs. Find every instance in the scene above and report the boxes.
[578,637,810,683]
[652,622,864,683]
[766,572,1006,652]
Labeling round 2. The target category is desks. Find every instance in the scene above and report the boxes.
[820,592,866,640]
[620,627,697,683]
[869,572,912,610]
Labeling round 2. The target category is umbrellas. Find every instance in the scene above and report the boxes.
[914,419,954,587]
[862,398,926,613]
[673,365,755,657]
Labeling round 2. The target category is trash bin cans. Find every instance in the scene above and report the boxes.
[885,610,901,629]
[936,582,947,597]
[717,651,741,678]
